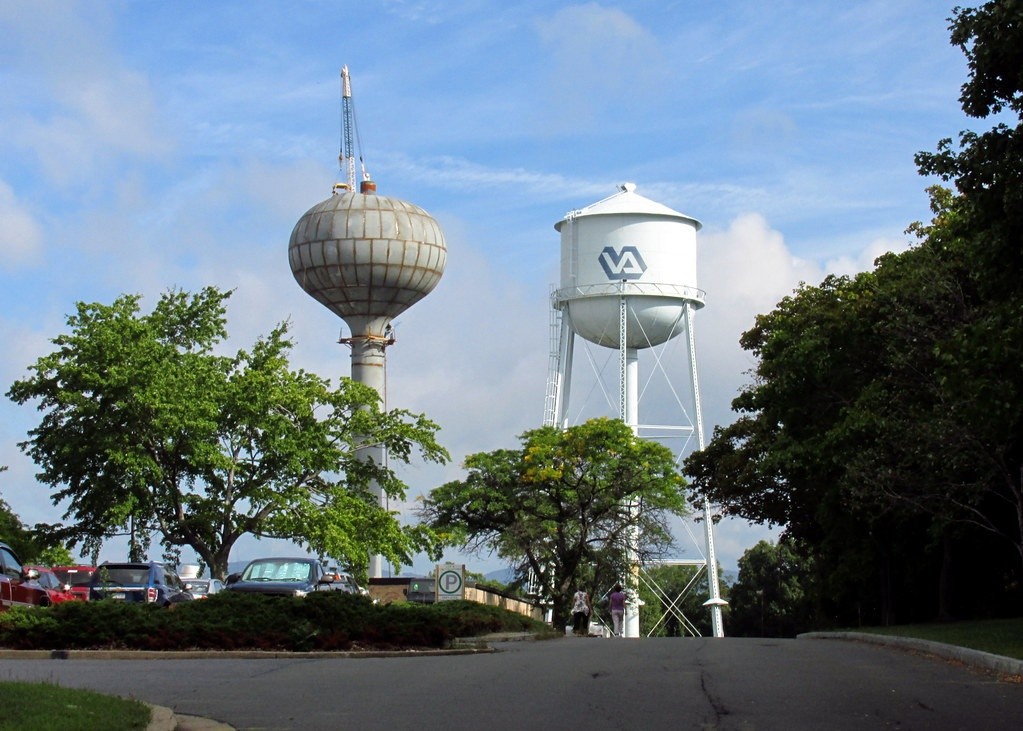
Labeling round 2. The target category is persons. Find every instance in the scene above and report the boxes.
[608,583,627,637]
[381,324,396,351]
[570,584,592,635]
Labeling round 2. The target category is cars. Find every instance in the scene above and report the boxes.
[0,535,436,614]
